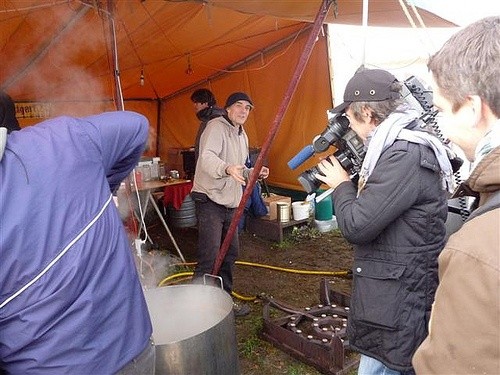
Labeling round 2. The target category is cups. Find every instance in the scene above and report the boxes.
[170,169,179,181]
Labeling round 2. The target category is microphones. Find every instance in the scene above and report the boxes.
[288,145,315,170]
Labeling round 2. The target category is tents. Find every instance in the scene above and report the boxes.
[0,0,462,196]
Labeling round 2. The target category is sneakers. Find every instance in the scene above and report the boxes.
[231,296,251,316]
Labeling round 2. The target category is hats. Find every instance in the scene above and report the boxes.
[329,69,400,114]
[226,92,254,111]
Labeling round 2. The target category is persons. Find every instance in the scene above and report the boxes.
[411,16,499,374]
[188,92,270,318]
[313,67,458,374]
[0,91,154,374]
[177,87,226,175]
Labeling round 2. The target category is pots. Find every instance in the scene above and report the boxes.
[129,274,239,375]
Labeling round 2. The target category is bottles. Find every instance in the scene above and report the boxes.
[314,188,334,221]
[130,158,166,188]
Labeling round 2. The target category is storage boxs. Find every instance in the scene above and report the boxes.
[261,196,291,221]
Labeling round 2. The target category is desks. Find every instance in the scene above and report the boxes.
[121,178,191,269]
